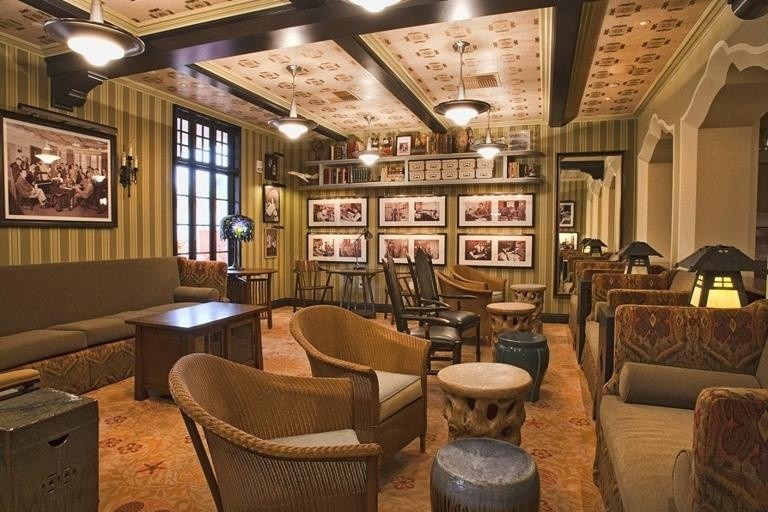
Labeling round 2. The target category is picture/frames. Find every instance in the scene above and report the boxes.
[307,193,535,269]
[558,200,579,256]
[0,109,116,228]
[263,153,281,258]
[396,135,412,155]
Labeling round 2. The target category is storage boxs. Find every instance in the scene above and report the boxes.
[408,158,494,181]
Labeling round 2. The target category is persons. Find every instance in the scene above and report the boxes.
[8,149,112,212]
[474,203,489,218]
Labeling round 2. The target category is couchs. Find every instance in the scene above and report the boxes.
[1,254,227,394]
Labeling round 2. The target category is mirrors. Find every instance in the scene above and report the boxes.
[551,150,624,298]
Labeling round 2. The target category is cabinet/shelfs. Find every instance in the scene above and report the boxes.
[298,150,546,191]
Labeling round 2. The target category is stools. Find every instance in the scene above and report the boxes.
[430,282,551,512]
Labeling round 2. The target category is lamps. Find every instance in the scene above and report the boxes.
[267,63,321,141]
[619,239,661,276]
[220,211,255,270]
[351,115,381,164]
[581,236,608,258]
[43,0,146,68]
[120,139,140,198]
[675,240,753,309]
[432,41,509,159]
